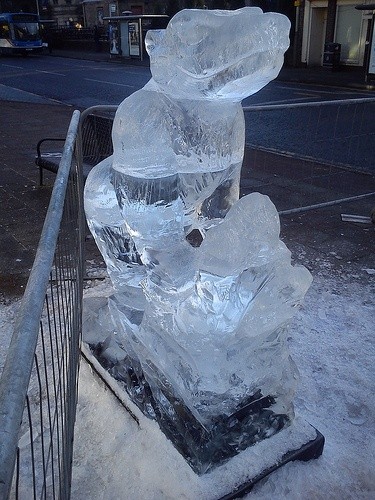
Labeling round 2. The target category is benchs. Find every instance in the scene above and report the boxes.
[34,105,118,182]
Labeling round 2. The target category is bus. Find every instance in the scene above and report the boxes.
[0,12,45,53]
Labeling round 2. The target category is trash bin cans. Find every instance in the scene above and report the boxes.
[322,43,342,72]
